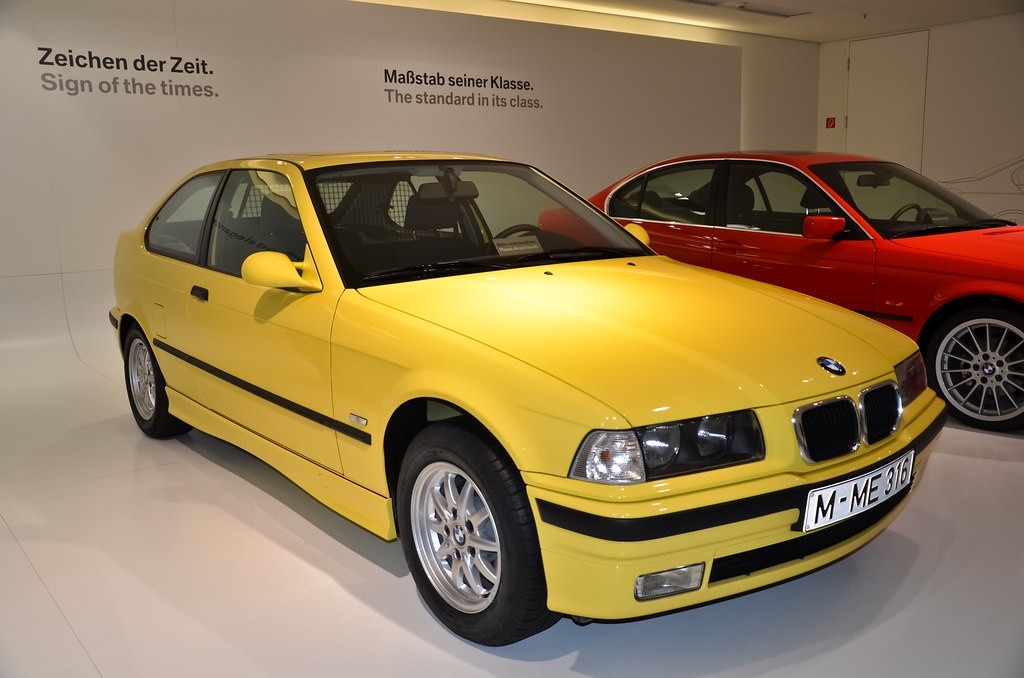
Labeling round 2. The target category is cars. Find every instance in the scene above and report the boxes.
[537,152,1024,433]
[107,152,948,648]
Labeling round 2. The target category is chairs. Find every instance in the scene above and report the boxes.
[237,196,306,262]
[735,184,767,227]
[800,187,835,232]
[393,196,482,269]
